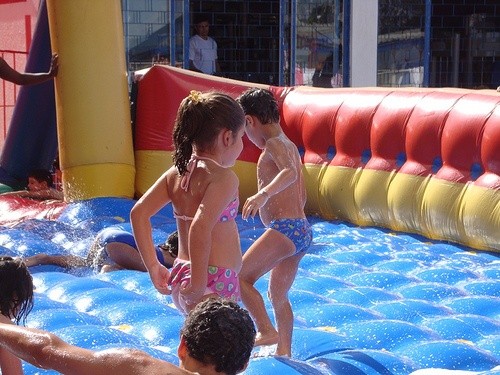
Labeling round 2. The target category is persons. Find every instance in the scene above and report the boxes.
[312,55,333,87]
[0,297,256,375]
[0,254,34,375]
[0,166,63,201]
[189,14,223,78]
[130,89,246,321]
[233,87,313,359]
[21,227,179,274]
